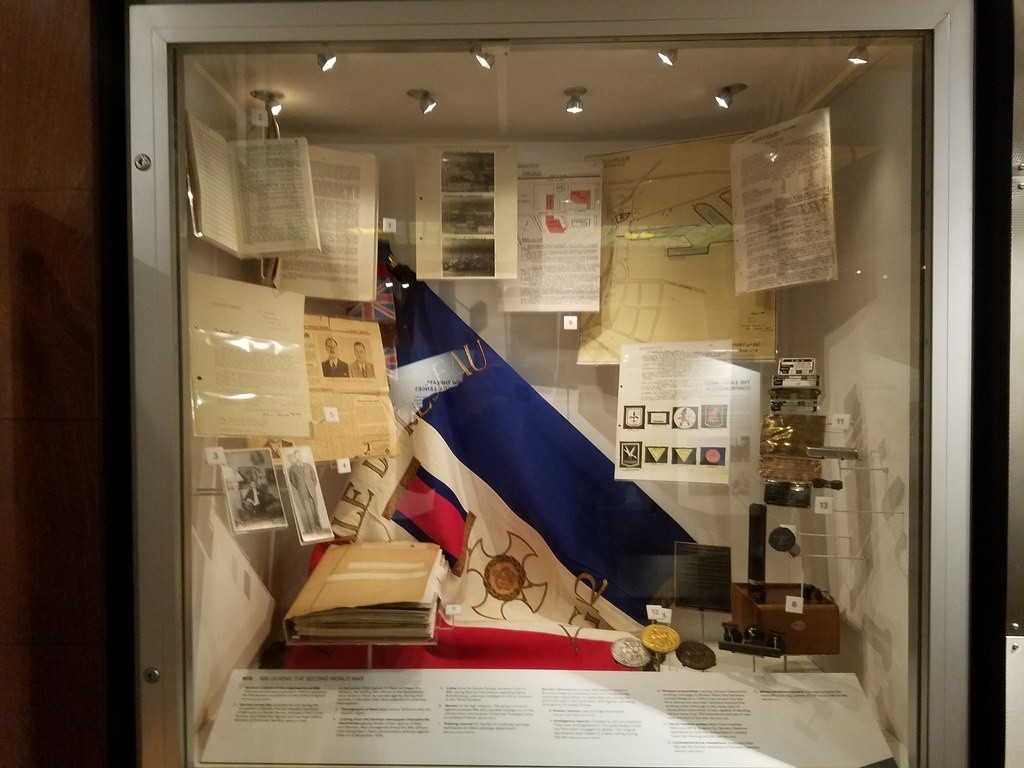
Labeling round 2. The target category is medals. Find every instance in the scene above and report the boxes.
[676,640,716,670]
[640,624,681,653]
[611,637,652,667]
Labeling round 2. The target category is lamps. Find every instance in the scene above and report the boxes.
[406,89,437,114]
[657,49,678,66]
[250,90,286,115]
[714,84,748,109]
[848,45,869,64]
[564,87,588,114]
[317,41,338,72]
[471,45,495,69]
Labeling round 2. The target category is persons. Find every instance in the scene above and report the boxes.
[322,338,374,378]
[245,479,267,519]
[289,449,325,535]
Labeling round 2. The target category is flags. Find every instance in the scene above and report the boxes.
[344,267,400,385]
[281,261,712,676]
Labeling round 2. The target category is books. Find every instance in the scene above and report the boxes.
[281,542,447,646]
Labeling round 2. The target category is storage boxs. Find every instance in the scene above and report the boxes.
[729,582,842,655]
